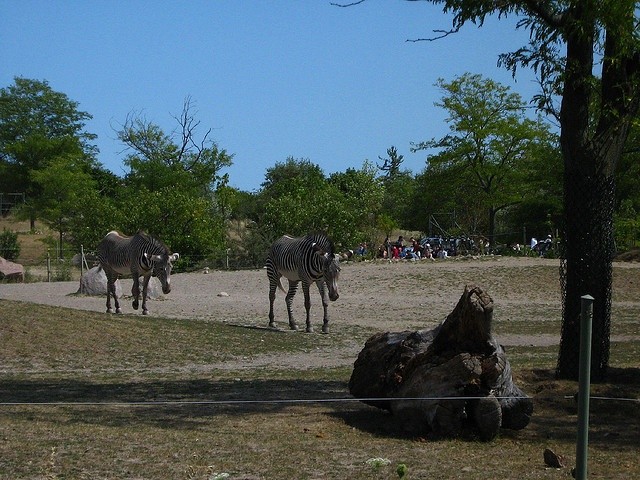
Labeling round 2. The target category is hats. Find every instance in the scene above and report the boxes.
[547,235,551,238]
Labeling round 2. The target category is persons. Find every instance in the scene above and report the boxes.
[544,234,552,251]
[356,242,364,254]
[478,237,484,256]
[484,238,490,255]
[378,235,474,260]
[363,242,367,254]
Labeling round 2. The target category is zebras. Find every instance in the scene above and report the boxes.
[264,231,342,334]
[93,229,182,316]
